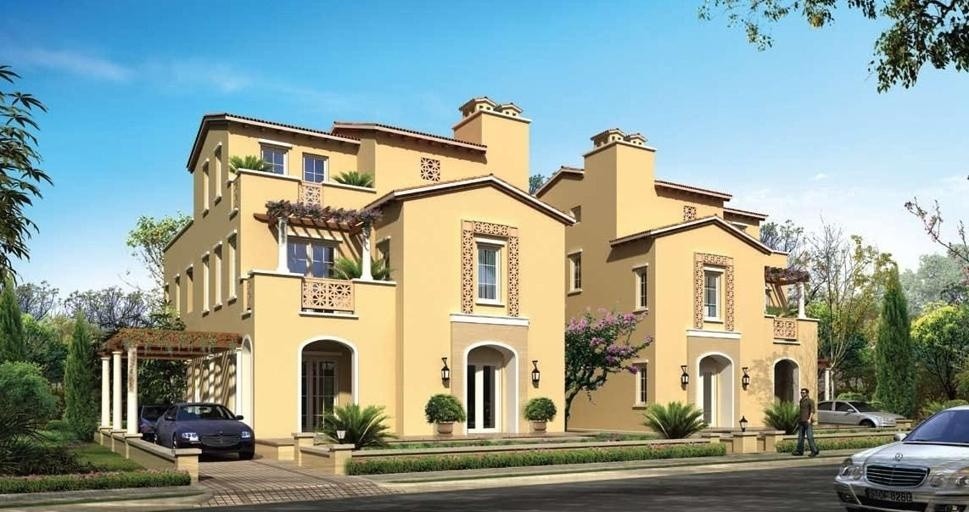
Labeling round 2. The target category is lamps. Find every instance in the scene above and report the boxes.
[742,367,750,385]
[532,360,540,383]
[441,357,449,382]
[681,365,689,384]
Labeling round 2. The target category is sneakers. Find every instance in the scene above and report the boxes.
[791,450,818,457]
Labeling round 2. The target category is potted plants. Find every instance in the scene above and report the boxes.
[424,393,467,434]
[521,397,558,431]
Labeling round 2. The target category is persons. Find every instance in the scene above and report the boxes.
[793,388,820,457]
